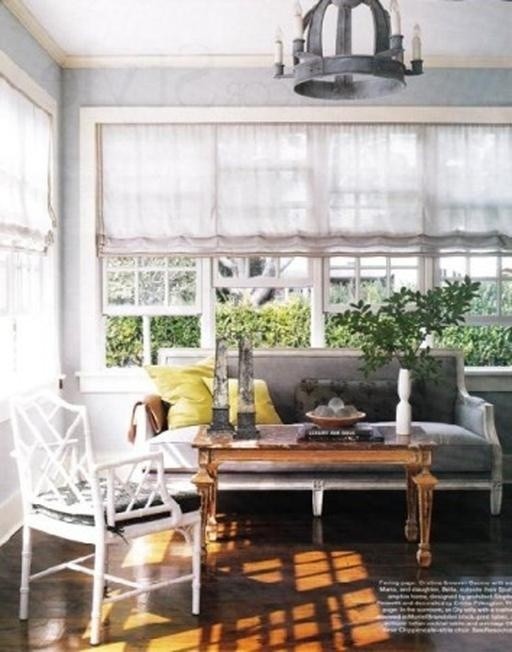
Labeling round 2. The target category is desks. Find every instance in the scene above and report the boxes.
[190,417,439,570]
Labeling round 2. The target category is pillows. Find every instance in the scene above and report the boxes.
[146,359,218,428]
[294,379,409,424]
[201,377,286,425]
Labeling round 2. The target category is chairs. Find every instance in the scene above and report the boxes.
[10,392,205,644]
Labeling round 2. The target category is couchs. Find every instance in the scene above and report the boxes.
[135,348,504,520]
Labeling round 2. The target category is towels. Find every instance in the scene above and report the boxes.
[126,394,166,444]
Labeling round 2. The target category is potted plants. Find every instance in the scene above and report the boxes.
[328,271,484,436]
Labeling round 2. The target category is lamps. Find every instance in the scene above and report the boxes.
[275,1,430,98]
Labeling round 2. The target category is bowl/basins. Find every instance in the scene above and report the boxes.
[304,410,367,429]
